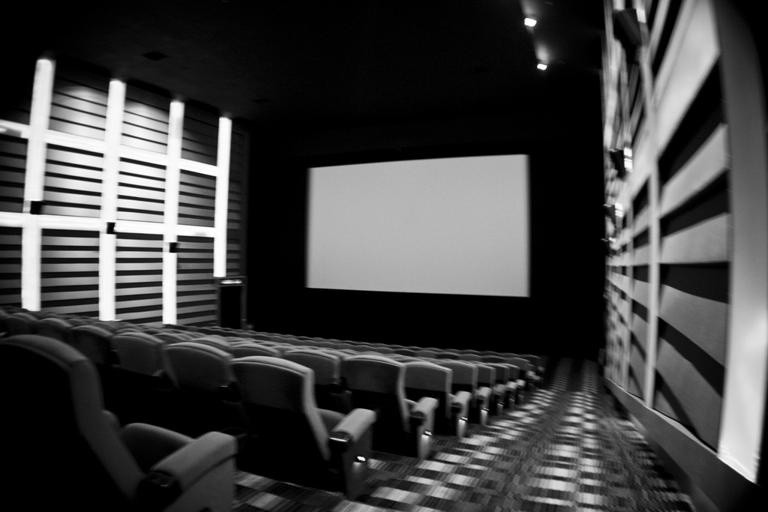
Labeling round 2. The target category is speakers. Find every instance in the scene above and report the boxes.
[608,149,625,179]
[170,243,177,252]
[604,204,615,226]
[30,201,42,214]
[217,275,244,330]
[107,223,114,233]
[600,239,609,252]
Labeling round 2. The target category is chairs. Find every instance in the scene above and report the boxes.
[0,302,550,512]
